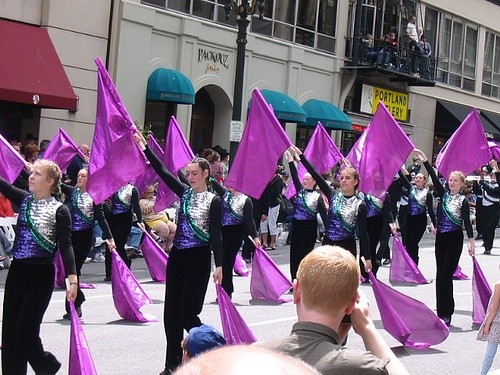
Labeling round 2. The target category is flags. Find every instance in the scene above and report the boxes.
[68,247,491,375]
[0,59,500,285]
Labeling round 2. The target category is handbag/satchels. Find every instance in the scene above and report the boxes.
[279,194,295,213]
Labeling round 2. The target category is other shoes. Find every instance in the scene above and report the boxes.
[104,278,112,283]
[484,250,491,254]
[286,241,291,245]
[63,308,83,319]
[442,317,452,324]
[475,235,482,239]
[383,259,391,264]
[316,239,321,242]
[216,293,232,301]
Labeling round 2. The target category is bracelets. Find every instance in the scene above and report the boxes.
[70,282,78,285]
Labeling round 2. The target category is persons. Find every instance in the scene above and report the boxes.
[171,345,323,375]
[131,133,222,375]
[182,324,226,365]
[0,134,500,328]
[362,15,430,76]
[268,246,409,375]
[476,283,500,375]
[0,161,78,375]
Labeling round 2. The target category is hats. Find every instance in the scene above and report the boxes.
[188,325,227,356]
[213,145,222,151]
[218,149,230,155]
[283,171,289,176]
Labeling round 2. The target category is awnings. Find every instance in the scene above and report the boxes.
[434,100,500,135]
[248,89,306,123]
[298,98,351,129]
[0,20,77,110]
[146,67,196,104]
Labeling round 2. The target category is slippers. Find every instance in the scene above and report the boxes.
[262,245,268,249]
[265,246,276,250]
